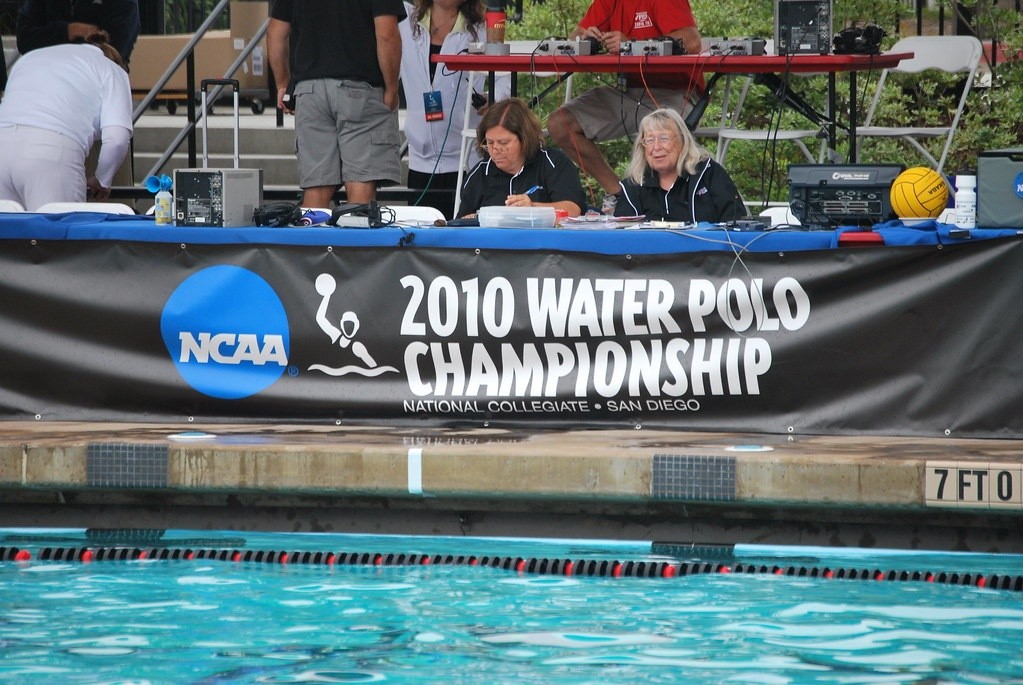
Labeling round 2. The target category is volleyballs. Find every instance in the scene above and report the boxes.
[889,166,949,217]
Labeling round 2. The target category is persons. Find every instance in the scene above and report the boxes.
[455,97,587,220]
[16,0,141,73]
[547,0,706,212]
[0,30,134,211]
[613,108,747,224]
[394,0,511,221]
[270,0,408,208]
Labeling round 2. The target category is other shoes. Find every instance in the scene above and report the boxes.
[600,192,619,216]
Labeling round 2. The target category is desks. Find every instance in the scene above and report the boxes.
[431,52,913,219]
[1,210,1022,439]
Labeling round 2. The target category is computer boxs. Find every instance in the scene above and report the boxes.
[774,0,833,55]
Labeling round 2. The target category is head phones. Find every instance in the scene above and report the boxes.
[326,201,387,229]
[252,200,303,227]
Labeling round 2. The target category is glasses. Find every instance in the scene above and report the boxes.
[481,138,518,154]
[641,133,678,148]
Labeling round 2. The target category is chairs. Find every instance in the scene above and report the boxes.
[450,35,981,221]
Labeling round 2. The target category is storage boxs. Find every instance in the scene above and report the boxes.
[973,148,1023,228]
[129,1,269,91]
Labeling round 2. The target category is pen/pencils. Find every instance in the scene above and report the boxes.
[525,185,539,195]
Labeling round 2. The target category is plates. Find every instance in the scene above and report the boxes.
[898,218,938,227]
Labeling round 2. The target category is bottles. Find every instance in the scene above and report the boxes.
[955,175,976,228]
[486,0,506,44]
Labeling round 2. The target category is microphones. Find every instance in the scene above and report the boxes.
[292,194,304,213]
